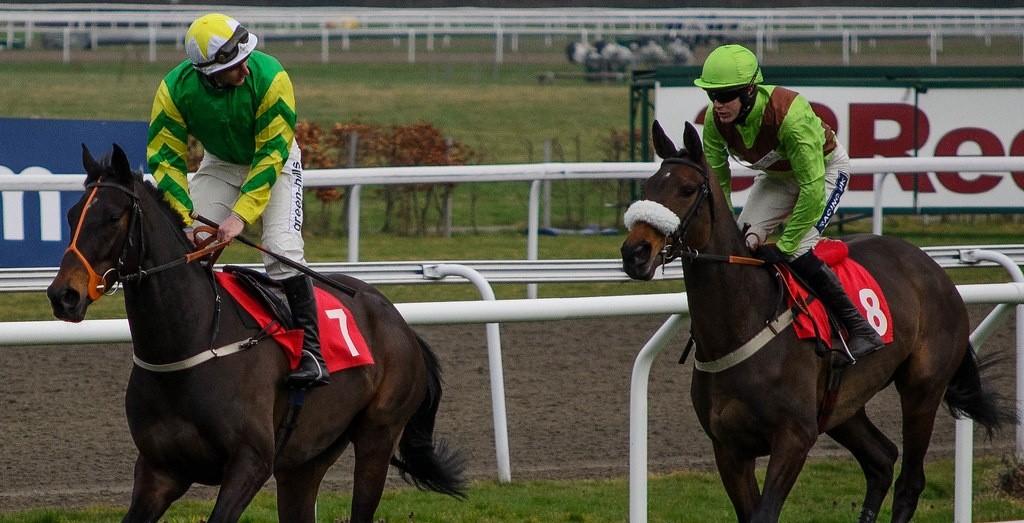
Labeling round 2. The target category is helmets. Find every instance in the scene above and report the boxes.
[185,11,259,76]
[692,44,765,90]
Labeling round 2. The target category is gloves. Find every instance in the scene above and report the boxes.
[754,245,784,264]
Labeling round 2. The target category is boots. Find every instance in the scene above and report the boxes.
[280,273,330,386]
[786,249,885,361]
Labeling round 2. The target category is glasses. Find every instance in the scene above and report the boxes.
[703,89,749,105]
[191,24,250,68]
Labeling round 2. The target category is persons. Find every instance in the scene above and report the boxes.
[146,13,329,386]
[693,44,885,358]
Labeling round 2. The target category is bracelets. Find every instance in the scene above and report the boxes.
[231,211,246,224]
[182,228,194,233]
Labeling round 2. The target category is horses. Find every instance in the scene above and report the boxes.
[618,118,1023,522]
[48,141,473,523]
